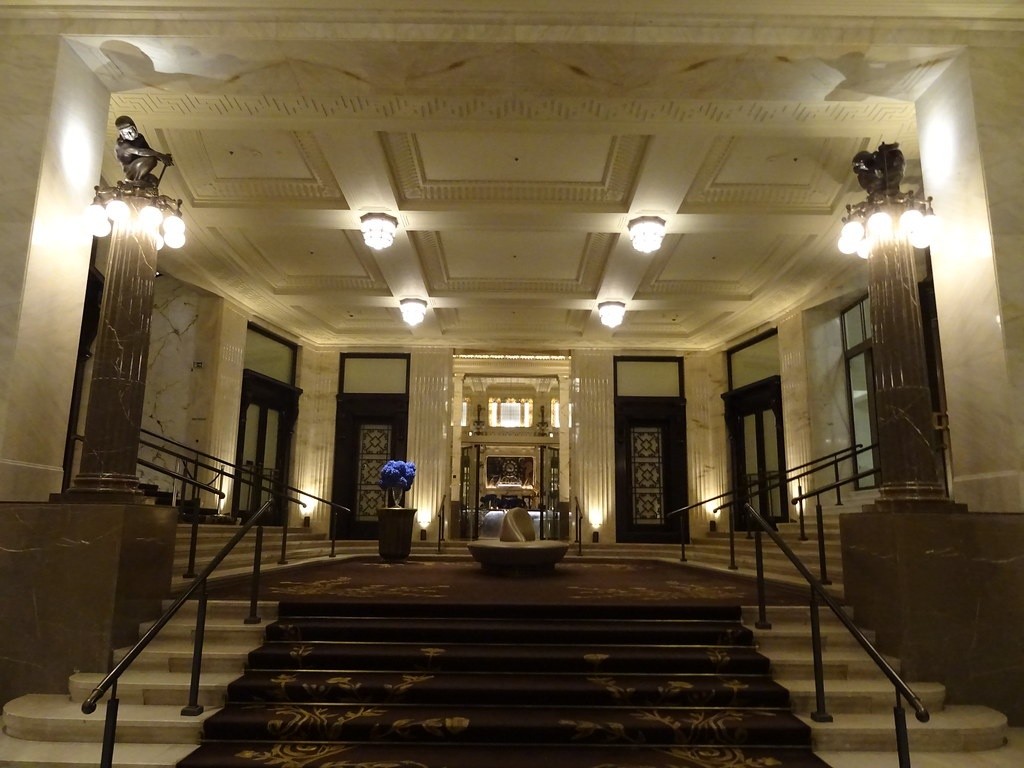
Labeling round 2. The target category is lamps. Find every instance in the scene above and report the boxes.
[304,516,311,529]
[360,213,398,251]
[834,144,941,254]
[399,298,428,326]
[629,216,668,254]
[593,531,599,543]
[710,521,717,531]
[598,301,626,327]
[421,530,426,540]
[92,170,186,248]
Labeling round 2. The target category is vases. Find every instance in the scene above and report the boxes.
[377,507,417,560]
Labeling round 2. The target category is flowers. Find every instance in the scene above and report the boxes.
[380,461,416,508]
